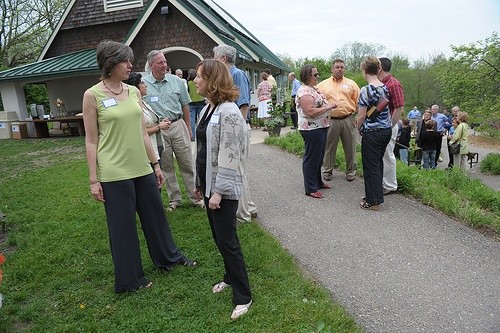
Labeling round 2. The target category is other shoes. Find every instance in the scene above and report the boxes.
[307,190,324,198]
[291,127,296,129]
[317,183,331,189]
[438,156,443,161]
[323,173,332,181]
[195,199,206,208]
[347,174,356,181]
[262,128,267,131]
[383,188,399,195]
[166,201,178,212]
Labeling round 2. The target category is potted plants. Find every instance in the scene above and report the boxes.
[265,84,287,138]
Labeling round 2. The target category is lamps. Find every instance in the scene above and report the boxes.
[160,6,170,14]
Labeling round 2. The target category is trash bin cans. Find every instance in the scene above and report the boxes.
[36,104,46,116]
[31,104,38,116]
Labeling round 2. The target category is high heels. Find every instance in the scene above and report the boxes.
[359,196,381,211]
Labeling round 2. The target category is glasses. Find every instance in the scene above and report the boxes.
[314,74,318,77]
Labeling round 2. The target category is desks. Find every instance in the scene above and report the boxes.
[19,115,85,136]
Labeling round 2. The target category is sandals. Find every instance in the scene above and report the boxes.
[179,257,197,267]
[127,279,152,291]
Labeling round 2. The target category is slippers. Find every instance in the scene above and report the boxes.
[230,298,253,320]
[212,281,232,294]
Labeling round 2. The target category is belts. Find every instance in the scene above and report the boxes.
[172,116,180,123]
[327,113,352,120]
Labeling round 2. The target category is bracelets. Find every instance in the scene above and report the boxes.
[152,161,158,166]
[326,107,328,110]
[89,180,98,185]
[158,125,160,131]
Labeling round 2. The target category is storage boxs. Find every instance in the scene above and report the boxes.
[11,123,27,139]
[63,127,79,137]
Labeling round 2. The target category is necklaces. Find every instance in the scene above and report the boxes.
[103,78,123,95]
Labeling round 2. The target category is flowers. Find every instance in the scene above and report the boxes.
[56,97,64,108]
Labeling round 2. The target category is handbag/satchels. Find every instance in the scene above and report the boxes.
[450,142,460,154]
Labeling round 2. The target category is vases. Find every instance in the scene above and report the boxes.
[57,108,68,117]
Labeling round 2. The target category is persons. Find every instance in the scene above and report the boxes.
[193,59,253,321]
[122,72,171,193]
[166,66,207,141]
[288,72,301,130]
[393,105,469,171]
[213,44,256,224]
[142,49,206,213]
[256,68,277,131]
[295,56,404,211]
[55,97,66,113]
[83,40,200,303]
